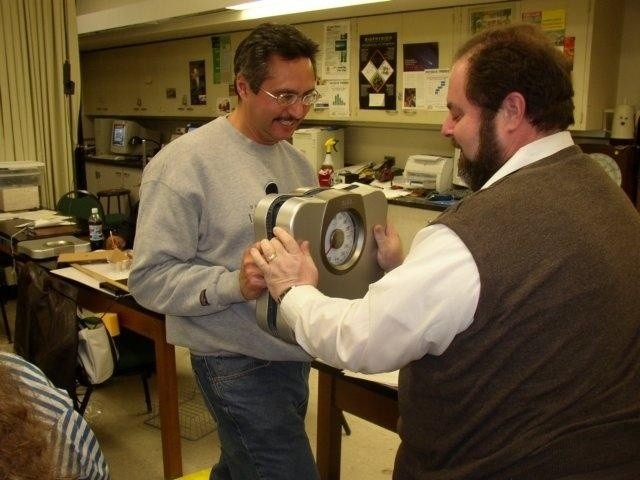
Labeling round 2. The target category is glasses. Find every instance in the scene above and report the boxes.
[257,86,323,107]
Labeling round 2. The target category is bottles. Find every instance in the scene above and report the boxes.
[87,207,105,250]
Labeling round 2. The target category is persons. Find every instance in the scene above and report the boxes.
[128,19,331,478]
[248,22,640,479]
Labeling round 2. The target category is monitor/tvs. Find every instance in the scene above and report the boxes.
[109,118,161,157]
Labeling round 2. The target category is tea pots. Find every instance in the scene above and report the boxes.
[602,97,640,139]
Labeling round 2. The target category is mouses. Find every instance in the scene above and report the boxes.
[115,155,125,160]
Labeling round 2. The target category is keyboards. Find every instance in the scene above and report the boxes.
[93,153,116,160]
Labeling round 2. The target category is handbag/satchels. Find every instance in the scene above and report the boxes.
[76,305,120,386]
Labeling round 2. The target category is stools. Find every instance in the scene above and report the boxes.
[97,187,132,226]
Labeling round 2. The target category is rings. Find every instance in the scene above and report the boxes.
[266,252,277,262]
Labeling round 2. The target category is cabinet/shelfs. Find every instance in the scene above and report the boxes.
[80,19,348,119]
[386,203,444,267]
[348,1,611,131]
[85,160,143,219]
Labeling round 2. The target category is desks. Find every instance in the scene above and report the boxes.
[311,358,399,480]
[0,207,182,480]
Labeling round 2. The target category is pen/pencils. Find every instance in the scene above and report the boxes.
[109,230,116,246]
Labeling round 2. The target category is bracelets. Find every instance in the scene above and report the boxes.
[277,280,315,305]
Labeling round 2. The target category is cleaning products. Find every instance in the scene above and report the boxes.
[317,137,340,187]
